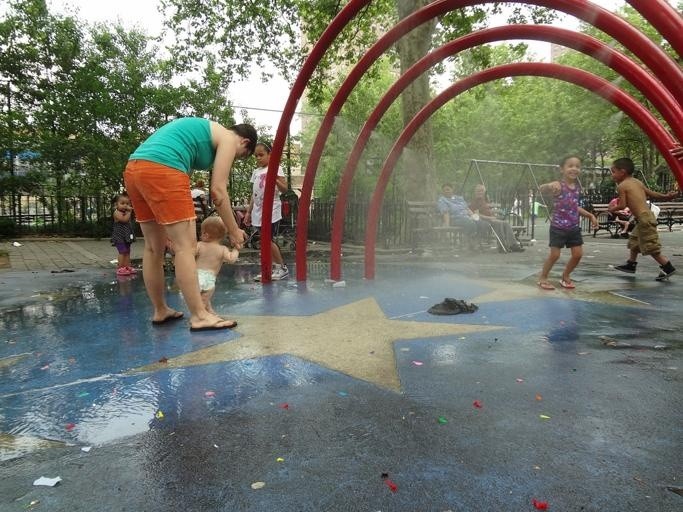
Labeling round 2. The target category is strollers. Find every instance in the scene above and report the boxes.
[243,186,304,255]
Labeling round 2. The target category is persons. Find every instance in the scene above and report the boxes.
[438,184,511,254]
[164,180,251,273]
[194,215,244,316]
[244,141,289,283]
[605,157,679,280]
[122,115,257,333]
[537,154,597,290]
[509,177,660,247]
[112,192,138,276]
[469,185,526,253]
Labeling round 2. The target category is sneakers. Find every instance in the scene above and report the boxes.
[614,259,638,273]
[499,244,524,252]
[117,265,138,276]
[655,260,678,279]
[253,261,289,280]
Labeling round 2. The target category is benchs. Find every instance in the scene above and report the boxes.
[589,202,682,239]
[404,200,527,256]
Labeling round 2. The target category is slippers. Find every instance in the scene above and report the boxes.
[537,282,556,289]
[152,311,184,325]
[190,320,237,332]
[559,279,575,288]
